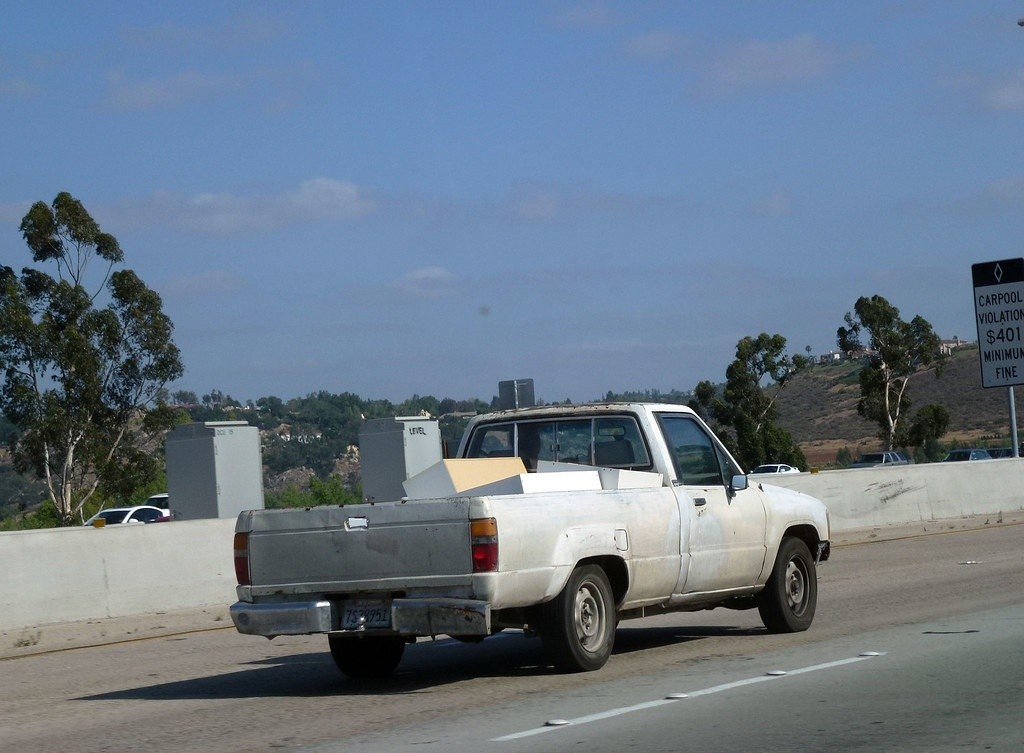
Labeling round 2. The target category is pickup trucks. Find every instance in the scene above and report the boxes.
[229,402,831,673]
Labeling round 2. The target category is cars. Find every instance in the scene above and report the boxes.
[851,451,909,468]
[940,448,1014,461]
[82,492,170,525]
[748,464,800,475]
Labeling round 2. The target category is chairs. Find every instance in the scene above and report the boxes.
[588,438,635,468]
[486,450,531,470]
